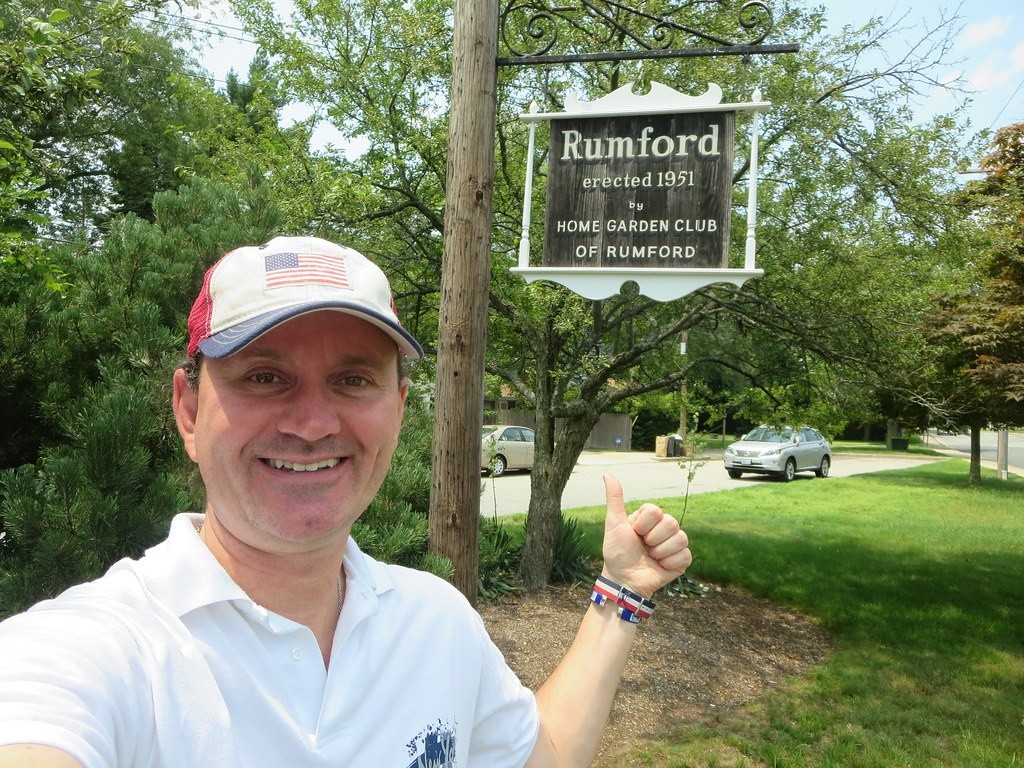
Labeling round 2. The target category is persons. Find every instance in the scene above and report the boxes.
[0,237,692,768]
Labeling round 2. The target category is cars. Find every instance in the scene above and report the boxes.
[482,424,556,477]
[722,424,832,483]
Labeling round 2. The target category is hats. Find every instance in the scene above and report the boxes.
[188,235,425,361]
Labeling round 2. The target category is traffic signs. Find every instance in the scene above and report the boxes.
[508,80,766,303]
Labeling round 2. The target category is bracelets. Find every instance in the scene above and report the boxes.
[591,575,656,623]
